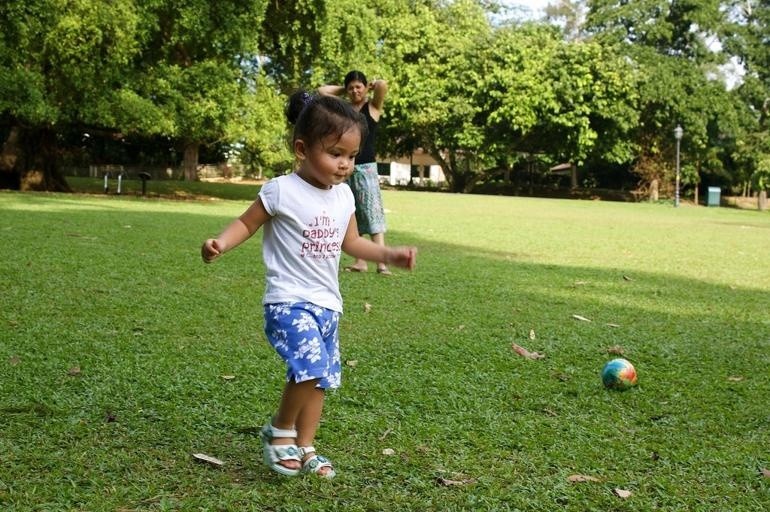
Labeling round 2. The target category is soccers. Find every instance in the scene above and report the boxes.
[601,359,638,392]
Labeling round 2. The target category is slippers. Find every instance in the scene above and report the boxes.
[378,267,392,275]
[344,266,368,272]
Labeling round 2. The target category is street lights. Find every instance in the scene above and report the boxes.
[673,123,685,206]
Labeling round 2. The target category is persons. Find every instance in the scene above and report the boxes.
[317,69,395,278]
[200,89,418,480]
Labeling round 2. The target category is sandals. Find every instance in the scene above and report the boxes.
[298,446,336,478]
[259,422,301,476]
